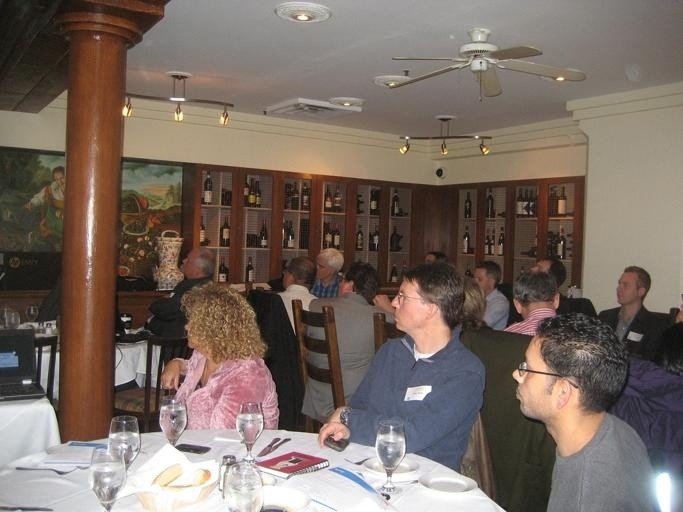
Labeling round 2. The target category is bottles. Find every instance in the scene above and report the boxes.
[390,226,399,252]
[218,256,230,283]
[485,226,503,255]
[465,264,471,278]
[462,226,470,253]
[515,185,568,217]
[246,256,254,283]
[533,228,566,259]
[392,188,401,217]
[200,215,380,251]
[391,265,398,283]
[204,168,379,215]
[463,192,472,218]
[486,189,494,217]
[219,454,242,498]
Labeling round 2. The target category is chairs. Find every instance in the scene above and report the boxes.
[0,275,193,466]
[649,308,680,333]
[374,313,558,512]
[292,299,346,432]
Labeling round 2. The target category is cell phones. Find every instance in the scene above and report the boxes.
[175,443,211,454]
[324,434,348,452]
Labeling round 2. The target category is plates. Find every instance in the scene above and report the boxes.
[364,456,420,477]
[257,474,313,512]
[166,466,219,489]
[417,472,479,494]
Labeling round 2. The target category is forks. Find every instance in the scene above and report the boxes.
[344,458,370,466]
[15,465,78,476]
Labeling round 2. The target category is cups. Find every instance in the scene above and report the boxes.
[225,462,263,512]
[4,306,61,337]
[120,313,132,334]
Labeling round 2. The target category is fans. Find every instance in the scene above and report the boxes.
[388,23,586,98]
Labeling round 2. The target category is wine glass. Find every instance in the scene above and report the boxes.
[236,401,264,464]
[90,445,127,511]
[376,418,406,494]
[158,394,186,446]
[107,416,141,471]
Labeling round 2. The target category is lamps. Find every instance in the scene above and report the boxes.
[118,71,232,126]
[397,115,493,156]
[274,1,332,24]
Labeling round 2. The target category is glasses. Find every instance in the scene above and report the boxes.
[518,362,578,388]
[398,293,420,305]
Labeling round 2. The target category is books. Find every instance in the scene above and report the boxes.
[251,451,329,480]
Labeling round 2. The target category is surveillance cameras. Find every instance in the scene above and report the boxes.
[435,167,447,177]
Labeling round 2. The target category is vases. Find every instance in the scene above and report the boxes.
[150,236,184,291]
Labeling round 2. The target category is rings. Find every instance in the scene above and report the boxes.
[159,385,164,389]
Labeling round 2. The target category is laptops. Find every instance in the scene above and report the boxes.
[0,329,45,402]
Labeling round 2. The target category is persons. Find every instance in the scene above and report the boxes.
[511,311,664,512]
[22,167,65,252]
[309,248,345,297]
[300,260,395,425]
[536,257,597,317]
[424,251,449,263]
[144,247,216,363]
[655,294,683,377]
[159,282,280,430]
[317,260,486,473]
[598,266,674,362]
[472,261,510,331]
[274,256,319,336]
[502,265,561,336]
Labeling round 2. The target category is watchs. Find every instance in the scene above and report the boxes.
[340,407,351,429]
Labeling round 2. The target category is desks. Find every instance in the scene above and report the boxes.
[0,427,507,512]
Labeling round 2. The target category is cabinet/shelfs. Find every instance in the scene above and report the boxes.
[179,162,586,299]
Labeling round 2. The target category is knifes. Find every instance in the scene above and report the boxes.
[0,506,54,512]
[256,439,280,458]
[270,438,291,454]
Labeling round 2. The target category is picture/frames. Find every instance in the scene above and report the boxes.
[0,145,183,276]
